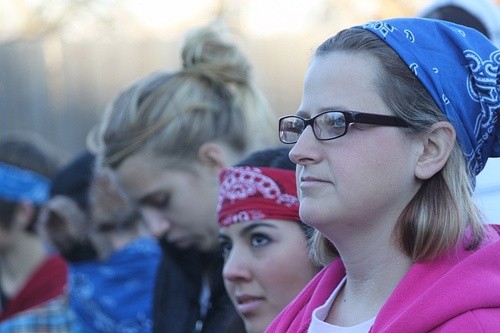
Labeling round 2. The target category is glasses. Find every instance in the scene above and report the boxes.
[279,110,411,143]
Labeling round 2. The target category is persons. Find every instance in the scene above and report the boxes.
[0,130,160,333]
[91,20,276,332]
[280,15,499,333]
[210,144,336,332]
